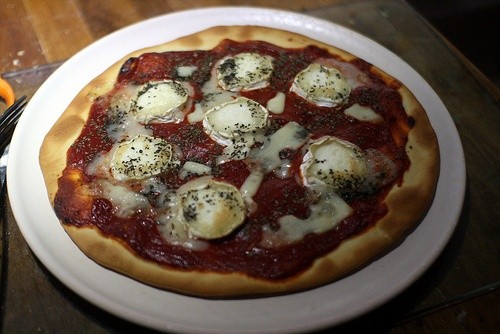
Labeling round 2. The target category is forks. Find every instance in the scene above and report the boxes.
[1,95,29,163]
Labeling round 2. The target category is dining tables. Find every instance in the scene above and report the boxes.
[1,2,500,333]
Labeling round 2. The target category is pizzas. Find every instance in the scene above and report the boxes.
[40,24,440,296]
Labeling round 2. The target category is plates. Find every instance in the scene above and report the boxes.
[6,6,469,333]
